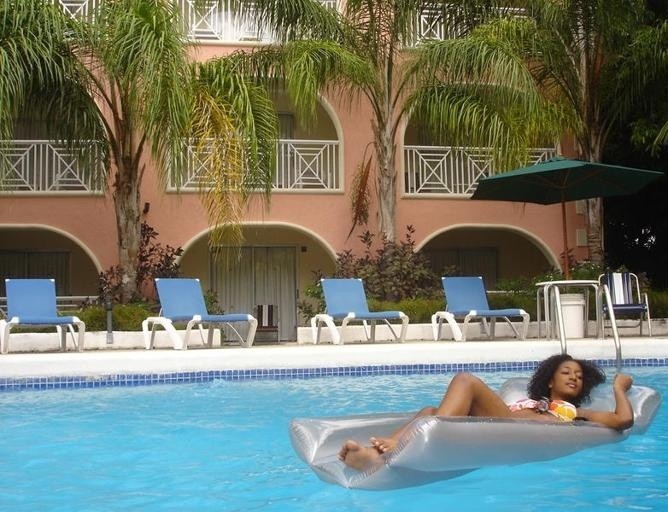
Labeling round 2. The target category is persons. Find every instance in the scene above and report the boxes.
[336,353,636,471]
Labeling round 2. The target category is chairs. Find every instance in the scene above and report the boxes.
[0,278,86,354]
[431,276,531,342]
[251,304,282,345]
[142,278,258,349]
[310,278,409,345]
[598,272,652,340]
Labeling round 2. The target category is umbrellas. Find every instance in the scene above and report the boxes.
[471,154,667,280]
